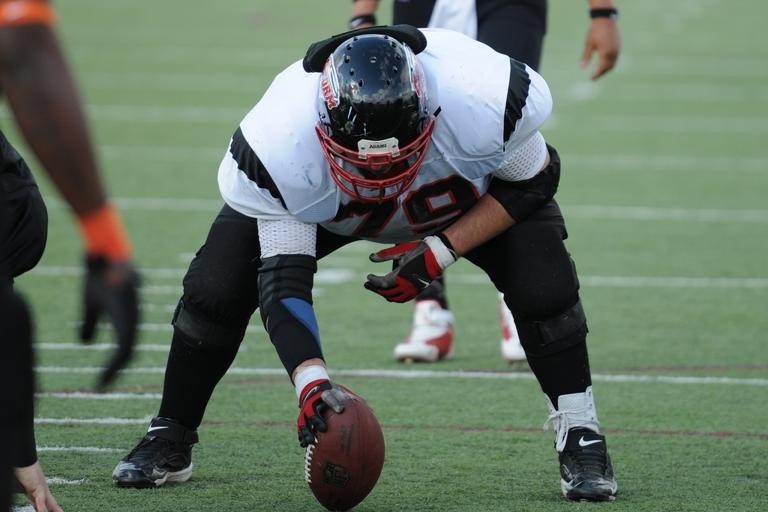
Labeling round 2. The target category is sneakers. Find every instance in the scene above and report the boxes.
[498,292,528,361]
[395,300,456,362]
[112,417,198,489]
[554,426,618,501]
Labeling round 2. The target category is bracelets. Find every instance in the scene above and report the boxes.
[296,362,326,397]
[350,13,374,31]
[81,198,126,265]
[588,6,618,19]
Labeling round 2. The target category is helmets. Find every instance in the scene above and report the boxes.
[315,33,435,203]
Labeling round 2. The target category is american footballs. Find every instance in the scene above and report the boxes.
[304,397,386,512]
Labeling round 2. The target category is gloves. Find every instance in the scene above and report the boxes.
[364,233,457,303]
[82,207,138,390]
[297,379,345,447]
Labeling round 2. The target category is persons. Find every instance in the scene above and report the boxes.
[109,24,622,502]
[3,284,65,510]
[349,2,624,360]
[2,3,141,510]
